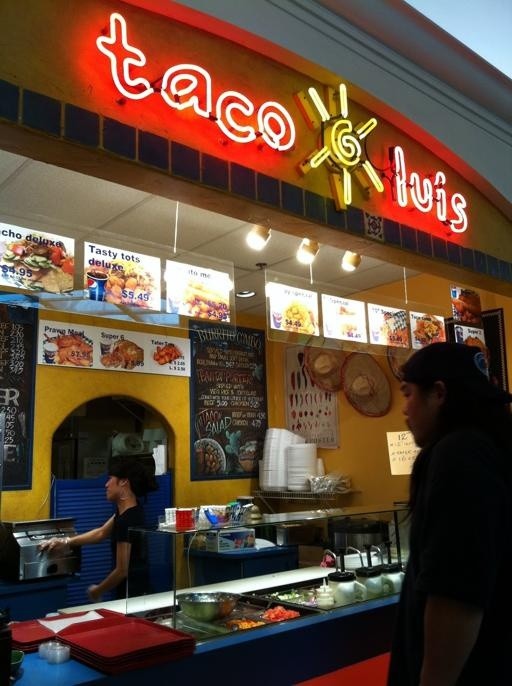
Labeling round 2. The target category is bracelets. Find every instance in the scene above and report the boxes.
[63,536,71,551]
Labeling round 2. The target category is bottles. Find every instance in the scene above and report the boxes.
[314,563,407,609]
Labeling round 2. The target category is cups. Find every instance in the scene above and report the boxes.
[86,270,109,302]
[43,343,59,364]
[100,340,112,355]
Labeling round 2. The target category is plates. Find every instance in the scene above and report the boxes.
[257,426,327,495]
[336,550,380,570]
[194,437,227,476]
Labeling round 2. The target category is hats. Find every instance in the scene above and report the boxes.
[387,346,418,381]
[303,338,358,392]
[341,349,391,417]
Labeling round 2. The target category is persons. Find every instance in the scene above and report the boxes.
[36,456,158,601]
[382,338,512,685]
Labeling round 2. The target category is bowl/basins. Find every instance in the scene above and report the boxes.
[174,591,243,623]
